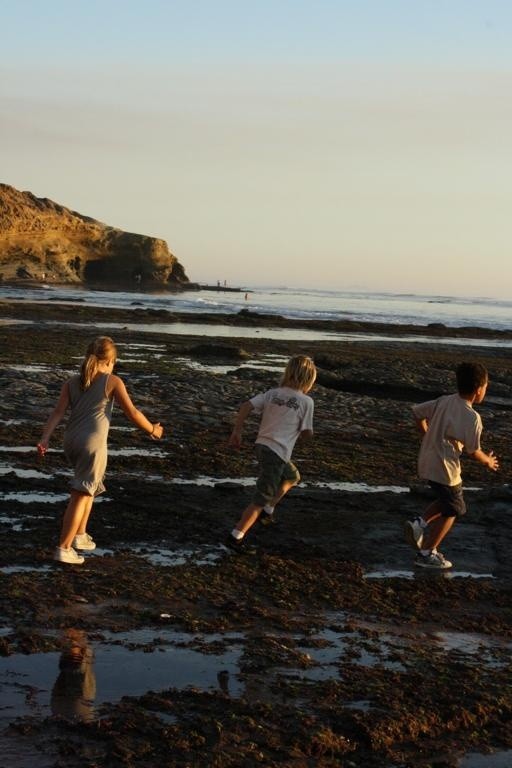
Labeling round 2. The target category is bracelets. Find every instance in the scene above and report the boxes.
[149,424,156,435]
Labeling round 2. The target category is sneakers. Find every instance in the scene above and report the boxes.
[226,536,248,553]
[406,516,425,551]
[53,546,84,564]
[258,510,273,526]
[413,549,453,569]
[74,534,96,550]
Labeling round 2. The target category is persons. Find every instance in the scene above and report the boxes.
[36,336,164,564]
[405,363,500,569]
[222,356,318,546]
